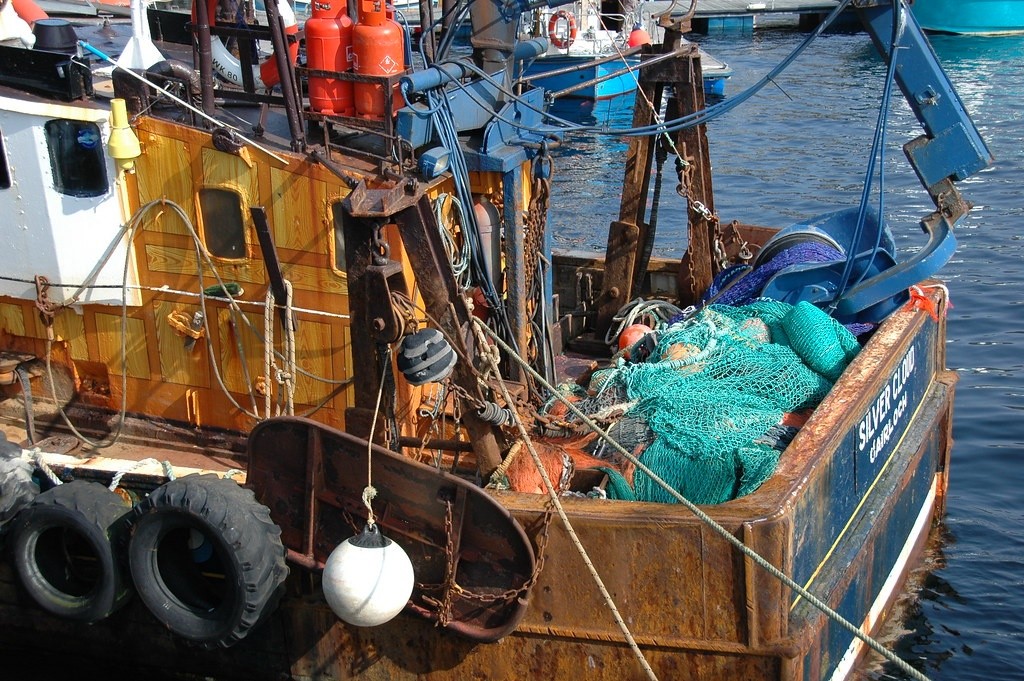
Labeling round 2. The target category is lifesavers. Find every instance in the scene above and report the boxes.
[548,9,576,48]
[128,473,292,650]
[10,479,133,635]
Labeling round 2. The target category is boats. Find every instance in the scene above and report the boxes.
[0,0,993,681]
